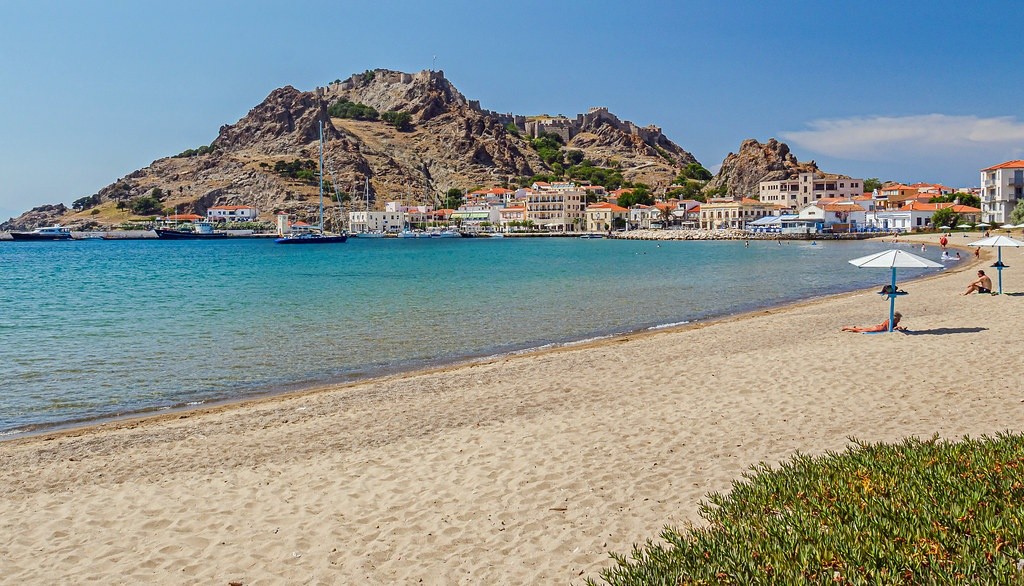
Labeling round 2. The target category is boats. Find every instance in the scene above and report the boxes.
[579,234,603,239]
[153,220,234,239]
[10,224,73,240]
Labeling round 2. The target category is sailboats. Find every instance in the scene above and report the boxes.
[273,120,504,244]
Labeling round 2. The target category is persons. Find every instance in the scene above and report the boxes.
[921,244,926,251]
[956,253,960,258]
[948,232,951,236]
[962,270,992,295]
[984,231,989,237]
[842,311,908,332]
[974,247,980,258]
[943,252,948,257]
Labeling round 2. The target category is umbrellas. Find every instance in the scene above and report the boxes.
[975,223,991,237]
[1016,223,1024,228]
[939,226,951,236]
[1000,224,1016,238]
[847,249,945,333]
[966,234,1024,294]
[956,224,972,236]
